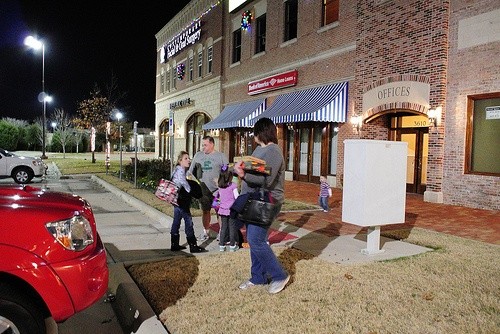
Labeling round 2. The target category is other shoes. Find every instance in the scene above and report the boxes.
[204,229,211,239]
[230,244,239,252]
[216,234,220,241]
[220,246,227,252]
[324,209,328,212]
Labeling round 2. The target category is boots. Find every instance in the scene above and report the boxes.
[186,235,205,253]
[170,234,187,251]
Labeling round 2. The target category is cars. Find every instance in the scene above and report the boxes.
[0,148,49,184]
[0,185,109,334]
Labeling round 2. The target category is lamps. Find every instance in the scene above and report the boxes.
[428,103,437,127]
[352,113,359,131]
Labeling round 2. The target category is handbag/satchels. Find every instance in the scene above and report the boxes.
[238,173,281,230]
[154,168,180,207]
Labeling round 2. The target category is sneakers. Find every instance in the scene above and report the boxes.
[269,274,291,294]
[239,281,263,289]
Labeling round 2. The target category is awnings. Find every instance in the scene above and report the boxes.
[202,98,267,129]
[250,81,348,126]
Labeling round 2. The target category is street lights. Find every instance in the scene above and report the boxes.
[23,35,51,160]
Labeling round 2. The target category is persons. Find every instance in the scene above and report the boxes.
[189,136,229,240]
[318,174,332,212]
[214,163,239,252]
[170,151,205,253]
[234,117,290,293]
